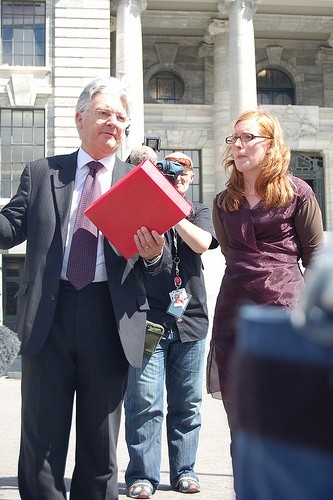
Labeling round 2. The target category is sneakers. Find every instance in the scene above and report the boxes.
[177,477,201,493]
[127,479,154,498]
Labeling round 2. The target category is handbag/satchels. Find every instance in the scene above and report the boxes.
[144,320,165,357]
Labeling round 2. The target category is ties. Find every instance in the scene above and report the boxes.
[65,161,104,291]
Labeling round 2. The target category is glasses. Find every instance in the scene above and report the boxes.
[224,133,272,144]
[95,108,129,122]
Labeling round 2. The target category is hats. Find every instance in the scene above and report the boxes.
[164,151,193,170]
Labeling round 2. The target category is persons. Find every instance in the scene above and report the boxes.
[0,77,219,500]
[173,294,182,305]
[205,114,322,430]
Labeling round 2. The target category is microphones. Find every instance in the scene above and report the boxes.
[131,145,157,167]
